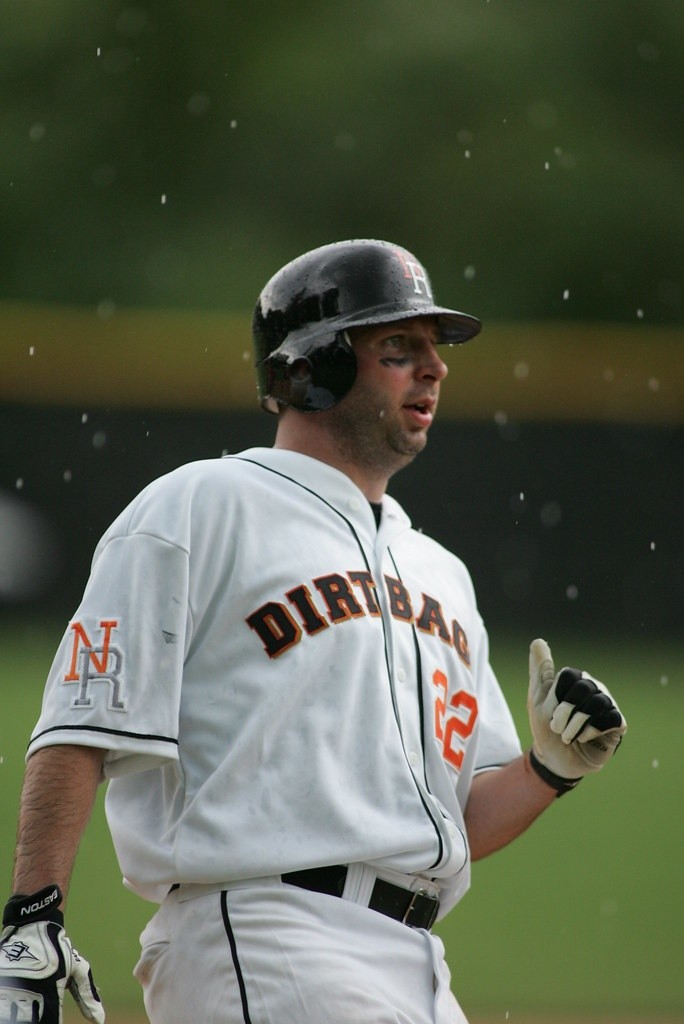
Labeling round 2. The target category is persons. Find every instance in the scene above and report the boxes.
[0,233,625,1024]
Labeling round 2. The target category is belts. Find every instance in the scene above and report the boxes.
[167,865,441,932]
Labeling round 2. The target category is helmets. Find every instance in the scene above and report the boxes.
[251,238,481,415]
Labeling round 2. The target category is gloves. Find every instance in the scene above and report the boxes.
[0,885,106,1023]
[527,638,627,796]
